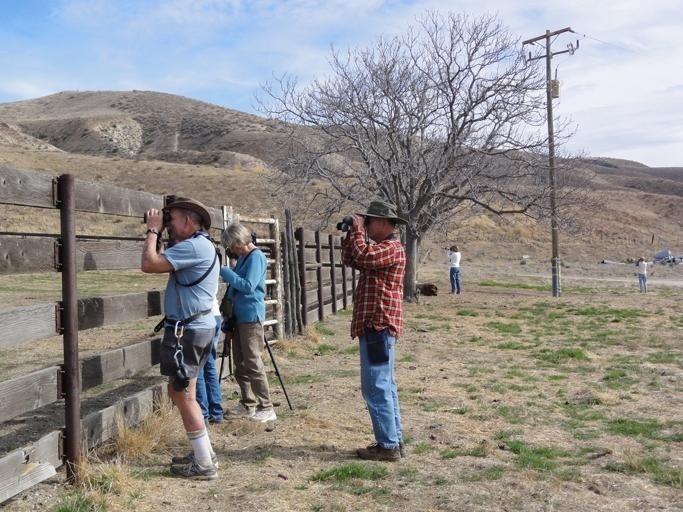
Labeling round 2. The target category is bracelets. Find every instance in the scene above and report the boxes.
[146,228,159,235]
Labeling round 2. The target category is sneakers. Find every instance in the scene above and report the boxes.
[250,407,278,423]
[170,453,219,467]
[169,459,219,481]
[224,404,257,419]
[355,442,406,461]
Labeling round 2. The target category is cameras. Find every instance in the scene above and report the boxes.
[221,316,239,333]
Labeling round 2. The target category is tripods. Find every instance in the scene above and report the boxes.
[217,312,293,412]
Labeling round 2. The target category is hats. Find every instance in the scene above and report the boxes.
[165,194,212,231]
[353,199,410,225]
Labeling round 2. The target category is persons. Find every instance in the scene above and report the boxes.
[219,225,277,423]
[636,257,647,293]
[448,246,461,294]
[339,199,406,461]
[141,193,220,480]
[195,293,223,424]
